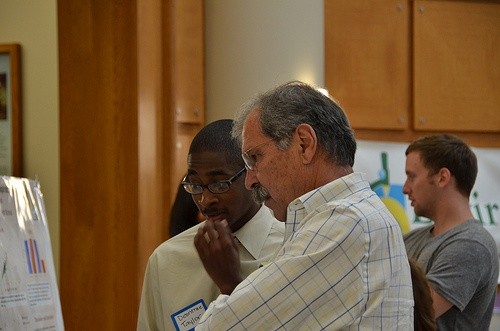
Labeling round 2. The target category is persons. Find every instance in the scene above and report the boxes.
[194,79,414,331]
[402,133,500,331]
[136,119,286,331]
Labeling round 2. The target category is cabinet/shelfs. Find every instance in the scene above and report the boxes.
[322,0,500,148]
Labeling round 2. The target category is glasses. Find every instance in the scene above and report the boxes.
[181,167,246,195]
[241,136,283,170]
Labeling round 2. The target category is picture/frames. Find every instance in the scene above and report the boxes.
[0,44,23,177]
